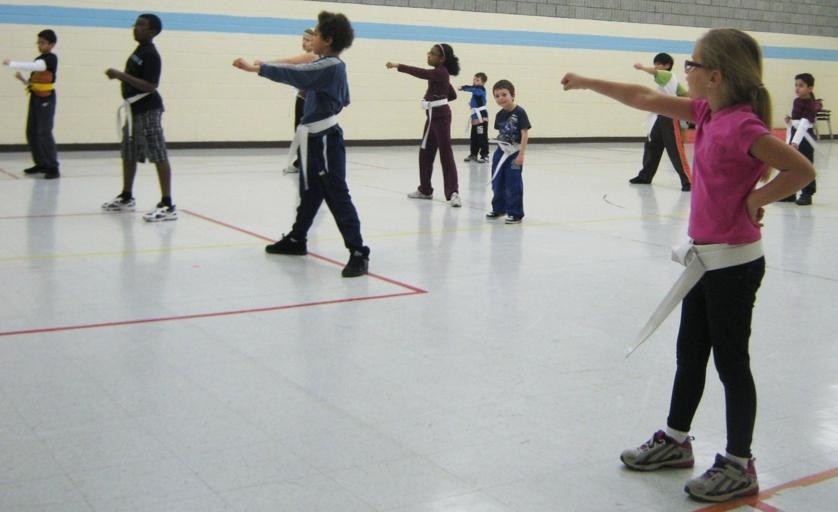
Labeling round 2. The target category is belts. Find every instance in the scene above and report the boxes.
[296,115,339,134]
[24,82,56,96]
[115,92,152,105]
[790,121,815,129]
[670,237,765,269]
[418,98,450,109]
[469,105,487,114]
[497,143,521,155]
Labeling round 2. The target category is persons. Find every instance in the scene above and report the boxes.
[101,14,178,222]
[458,72,489,162]
[629,53,693,191]
[776,74,822,205]
[387,43,463,208]
[486,80,532,225]
[561,28,816,502]
[232,11,369,276]
[3,30,60,178]
[253,28,322,173]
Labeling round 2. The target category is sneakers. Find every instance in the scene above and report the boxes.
[620,429,695,471]
[341,246,370,277]
[24,166,46,173]
[450,192,463,207]
[505,215,523,224]
[100,197,137,213]
[680,183,692,192]
[486,209,507,220]
[795,194,813,205]
[266,236,308,256]
[478,157,490,163]
[464,154,477,162]
[628,176,653,185]
[684,453,760,504]
[45,168,60,179]
[142,204,177,221]
[407,189,434,200]
